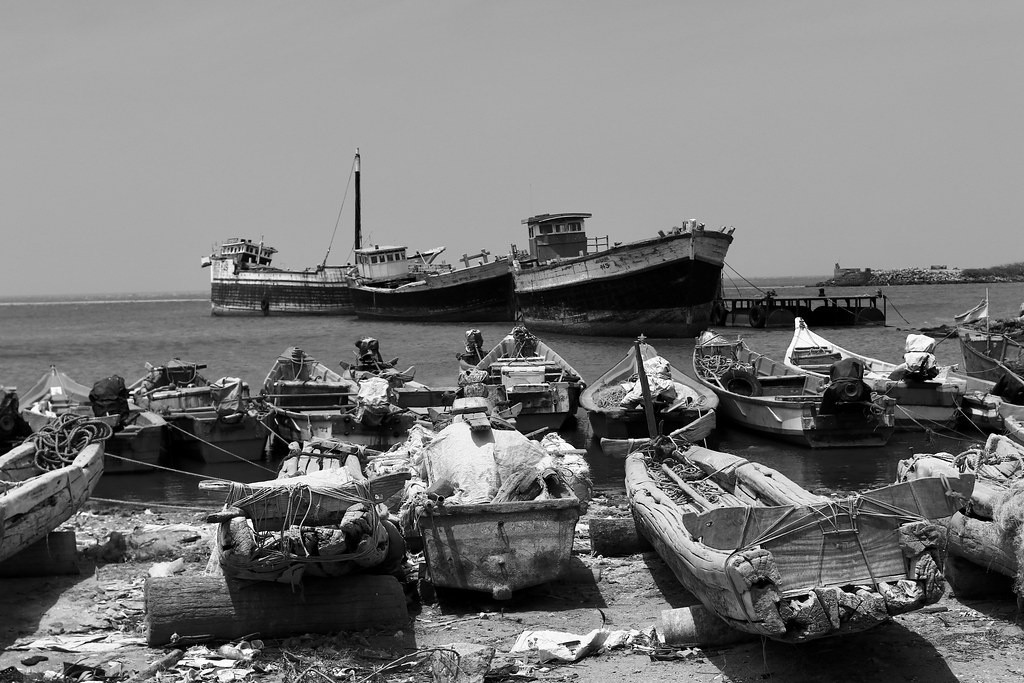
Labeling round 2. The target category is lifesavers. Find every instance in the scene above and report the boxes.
[720,370,763,398]
[261,298,269,310]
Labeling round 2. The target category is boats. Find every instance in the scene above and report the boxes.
[691,326,895,447]
[453,321,587,435]
[339,336,462,437]
[344,242,517,321]
[124,357,278,464]
[258,344,384,457]
[17,363,173,474]
[892,412,1023,589]
[782,316,968,436]
[621,431,953,646]
[0,414,113,571]
[492,208,735,334]
[381,396,591,600]
[580,332,719,463]
[822,260,891,285]
[952,285,1024,401]
[197,428,413,578]
[198,145,447,314]
